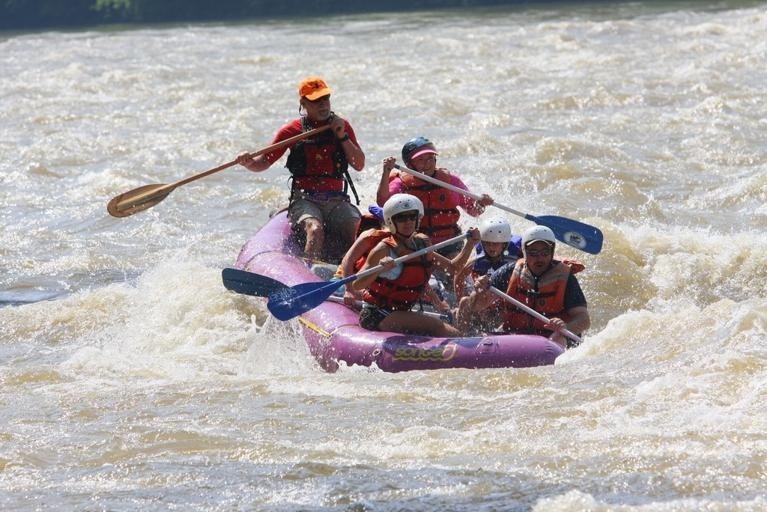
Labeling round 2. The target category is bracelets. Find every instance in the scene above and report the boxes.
[338,134,350,142]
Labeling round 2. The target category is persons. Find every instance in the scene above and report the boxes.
[377,136,495,281]
[469,224,590,348]
[329,227,391,306]
[351,192,481,338]
[452,213,518,332]
[235,78,365,256]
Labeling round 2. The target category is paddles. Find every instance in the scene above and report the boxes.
[107,118,341,218]
[267,229,473,322]
[394,164,603,255]
[222,267,452,323]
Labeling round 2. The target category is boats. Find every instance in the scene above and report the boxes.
[232,206,566,376]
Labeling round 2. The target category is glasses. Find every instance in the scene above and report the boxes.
[395,214,418,222]
[526,249,552,256]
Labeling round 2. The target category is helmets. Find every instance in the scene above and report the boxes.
[521,225,555,259]
[383,194,424,234]
[402,136,432,163]
[479,215,511,249]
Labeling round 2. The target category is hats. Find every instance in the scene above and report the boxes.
[410,145,439,160]
[526,239,554,248]
[298,76,332,101]
